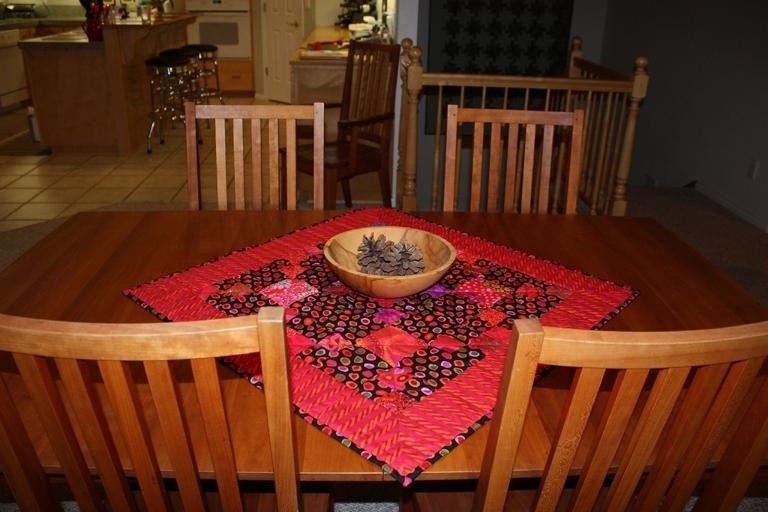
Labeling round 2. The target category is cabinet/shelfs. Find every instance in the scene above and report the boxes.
[290,24,389,202]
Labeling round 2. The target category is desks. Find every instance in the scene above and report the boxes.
[117,13,207,30]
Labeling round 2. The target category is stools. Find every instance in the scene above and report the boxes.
[142,44,227,153]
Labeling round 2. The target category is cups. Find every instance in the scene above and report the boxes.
[140,5,151,25]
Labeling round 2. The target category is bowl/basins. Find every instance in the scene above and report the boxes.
[323,225,456,301]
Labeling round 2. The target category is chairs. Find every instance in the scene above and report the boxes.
[278,39,402,210]
